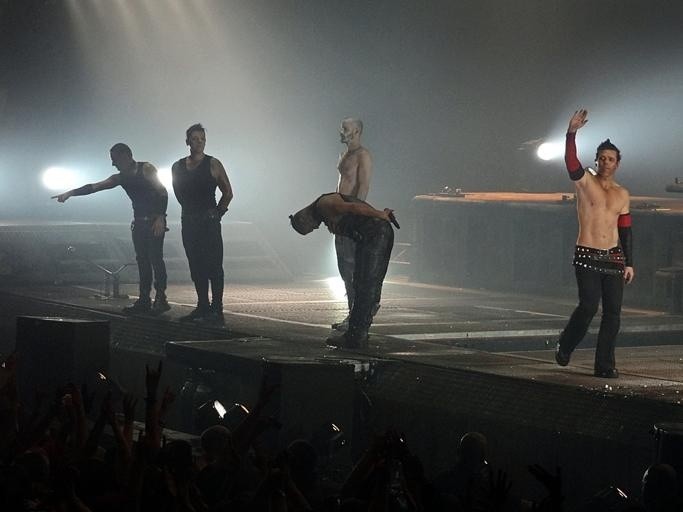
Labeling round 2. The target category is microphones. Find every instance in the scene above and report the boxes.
[145,224,169,231]
[383,207,401,230]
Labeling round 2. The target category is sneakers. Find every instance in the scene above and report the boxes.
[592,361,620,378]
[121,301,224,325]
[554,341,571,367]
[325,320,369,349]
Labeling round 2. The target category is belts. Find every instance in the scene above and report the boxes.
[576,245,622,257]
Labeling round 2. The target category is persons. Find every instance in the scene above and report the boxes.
[0,332,682,512]
[554,108,634,377]
[287,192,399,349]
[170,122,233,324]
[328,117,372,331]
[51,142,172,317]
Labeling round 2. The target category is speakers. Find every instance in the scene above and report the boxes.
[164,336,355,455]
[14,315,112,421]
